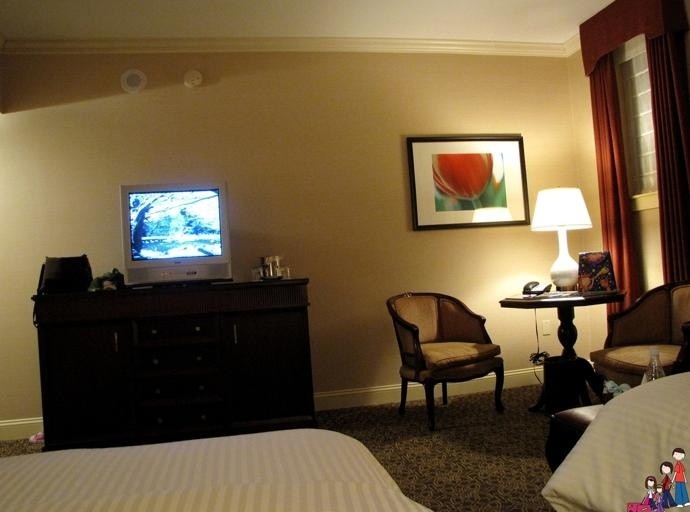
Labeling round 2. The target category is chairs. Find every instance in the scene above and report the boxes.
[388,290,504,428]
[590,277,688,387]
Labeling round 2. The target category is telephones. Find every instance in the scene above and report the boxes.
[522,282,552,295]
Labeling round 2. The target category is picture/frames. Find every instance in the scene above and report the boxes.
[407,134,531,231]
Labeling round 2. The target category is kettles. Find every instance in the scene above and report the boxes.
[260,254,283,279]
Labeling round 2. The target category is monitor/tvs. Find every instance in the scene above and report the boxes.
[120,180,231,285]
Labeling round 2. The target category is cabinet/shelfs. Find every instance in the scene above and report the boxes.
[31,278,316,449]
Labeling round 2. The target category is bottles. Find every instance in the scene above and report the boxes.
[645,346,665,382]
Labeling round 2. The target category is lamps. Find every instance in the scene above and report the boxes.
[529,188,593,290]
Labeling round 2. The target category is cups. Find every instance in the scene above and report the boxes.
[251,265,292,280]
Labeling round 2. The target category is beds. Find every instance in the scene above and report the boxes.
[544,369,689,512]
[0,426,424,512]
[500,291,628,417]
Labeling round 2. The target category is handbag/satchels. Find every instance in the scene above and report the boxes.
[38,255,93,294]
